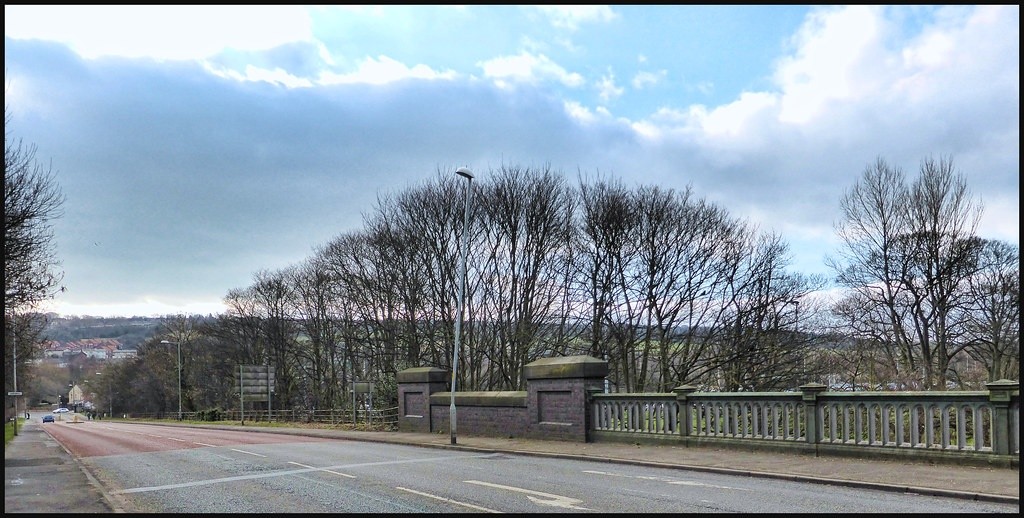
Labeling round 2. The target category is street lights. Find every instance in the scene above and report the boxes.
[69,384,76,413]
[445,169,477,443]
[58,394,61,408]
[96,372,113,418]
[160,340,183,421]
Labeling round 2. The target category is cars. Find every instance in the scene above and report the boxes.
[43,415,54,423]
[53,408,69,414]
